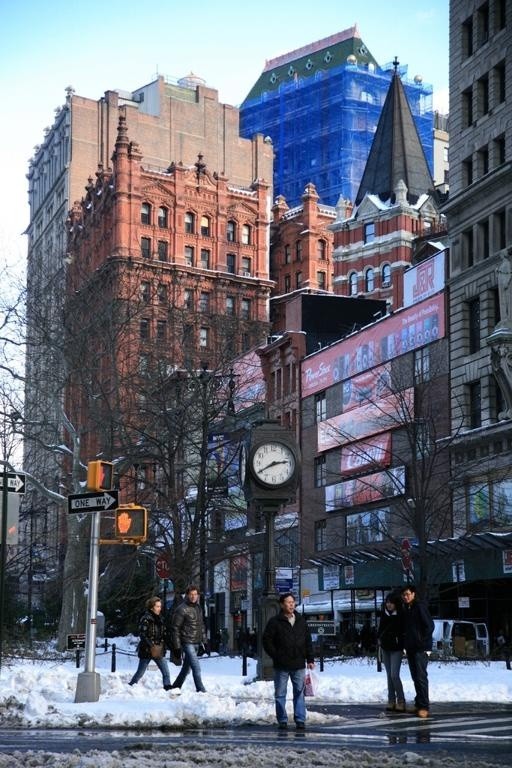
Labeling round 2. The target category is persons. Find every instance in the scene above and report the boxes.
[261,591,316,731]
[129,596,175,691]
[216,621,378,653]
[398,585,435,719]
[376,592,407,713]
[170,585,208,693]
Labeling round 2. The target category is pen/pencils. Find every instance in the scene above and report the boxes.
[432,619,490,661]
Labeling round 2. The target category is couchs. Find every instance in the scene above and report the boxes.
[87,460,114,492]
[114,504,148,542]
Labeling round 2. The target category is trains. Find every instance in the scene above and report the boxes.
[155,553,173,579]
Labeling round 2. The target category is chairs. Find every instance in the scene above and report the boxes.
[278,722,286,728]
[296,722,304,728]
[386,703,405,712]
[419,709,427,717]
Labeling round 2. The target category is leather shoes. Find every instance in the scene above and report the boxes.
[198,646,204,656]
[173,648,181,659]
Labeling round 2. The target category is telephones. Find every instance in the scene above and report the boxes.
[68,489,119,514]
[0,471,26,496]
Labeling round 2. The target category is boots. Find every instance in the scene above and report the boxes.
[311,633,341,659]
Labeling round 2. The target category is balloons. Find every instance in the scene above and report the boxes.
[248,436,298,490]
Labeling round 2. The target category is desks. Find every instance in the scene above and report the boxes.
[195,359,241,653]
